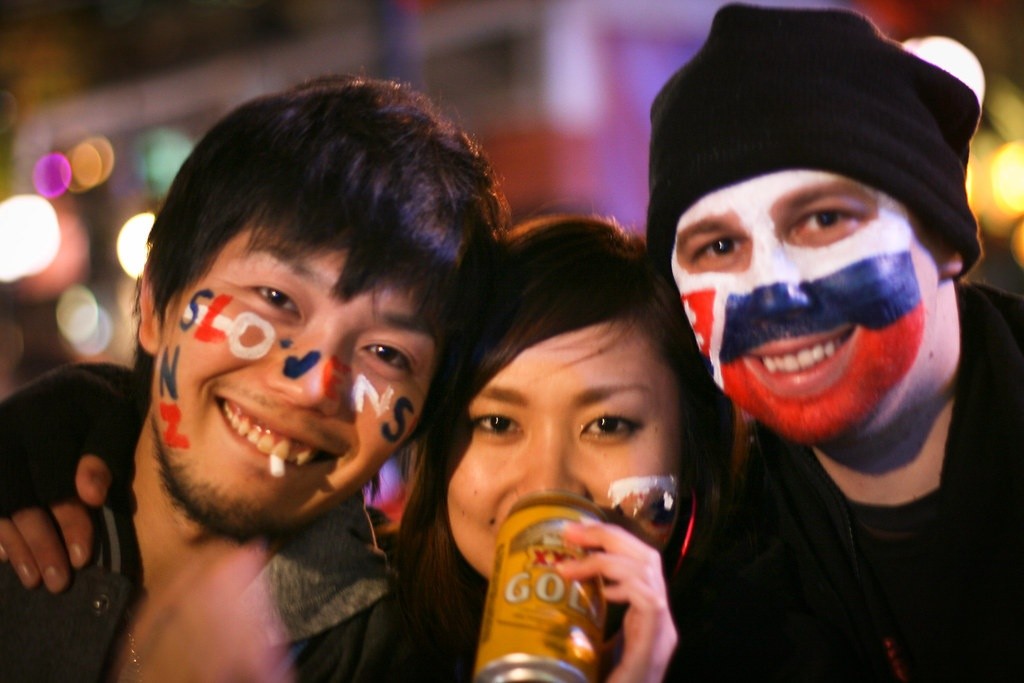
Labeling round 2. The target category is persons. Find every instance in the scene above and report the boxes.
[0,208,841,683]
[0,70,509,683]
[641,5,1024,683]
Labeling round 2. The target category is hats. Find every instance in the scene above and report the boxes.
[645,2,981,289]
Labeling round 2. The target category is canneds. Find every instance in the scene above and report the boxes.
[472,496,609,682]
